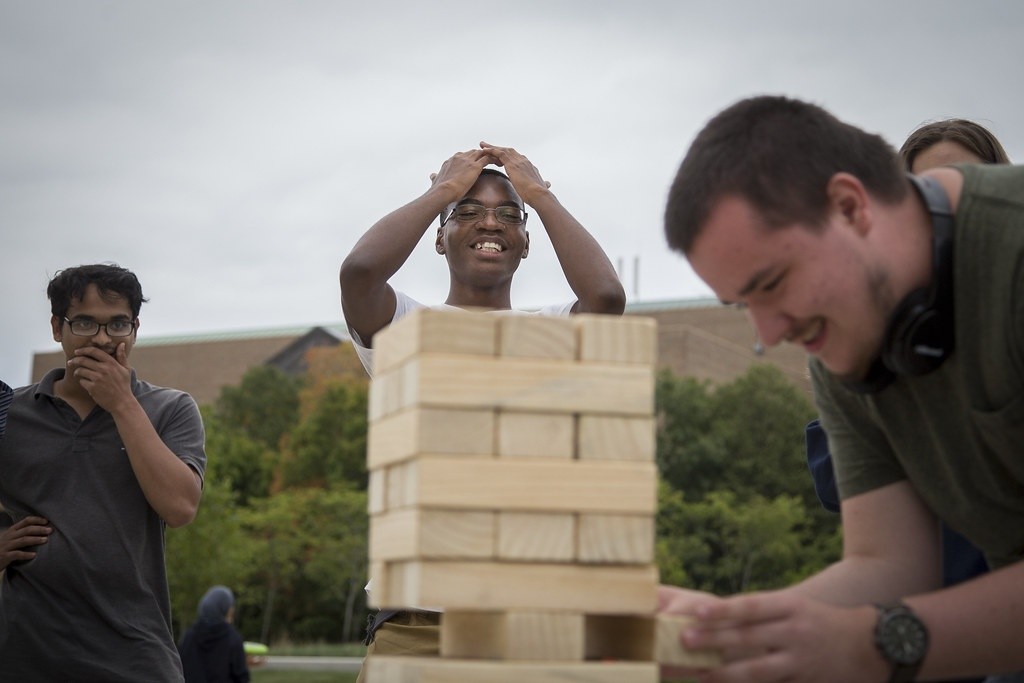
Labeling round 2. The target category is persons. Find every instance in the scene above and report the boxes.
[337,140,627,683]
[898,118,1009,175]
[1,265,209,682]
[177,585,254,682]
[659,95,1024,683]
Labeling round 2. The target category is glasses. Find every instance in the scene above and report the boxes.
[441,203,529,225]
[63,316,137,337]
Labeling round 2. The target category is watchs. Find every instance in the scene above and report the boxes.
[871,597,932,683]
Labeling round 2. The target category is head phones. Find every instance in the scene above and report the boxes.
[840,172,955,395]
